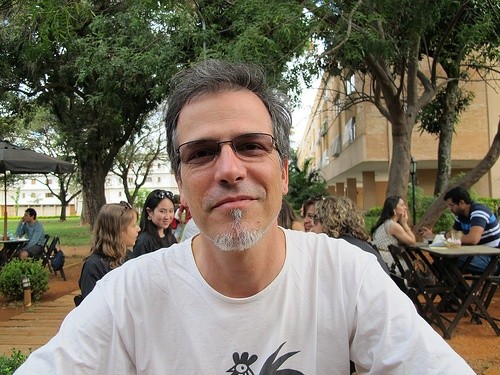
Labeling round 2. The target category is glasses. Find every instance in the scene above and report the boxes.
[119,201,133,217]
[313,215,321,226]
[174,132,284,166]
[149,191,174,202]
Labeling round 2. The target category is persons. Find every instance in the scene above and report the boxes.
[371,196,460,311]
[171,193,199,244]
[80,203,141,300]
[133,189,178,257]
[13,61,477,375]
[12,208,45,257]
[419,187,500,313]
[277,199,306,232]
[304,198,326,232]
[313,194,390,276]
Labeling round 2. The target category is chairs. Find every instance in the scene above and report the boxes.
[34,234,66,281]
[388,244,500,329]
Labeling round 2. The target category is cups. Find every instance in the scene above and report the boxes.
[451,231,461,248]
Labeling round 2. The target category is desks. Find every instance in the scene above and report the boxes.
[420,246,500,339]
[0,238,30,267]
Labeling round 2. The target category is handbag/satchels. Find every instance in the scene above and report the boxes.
[51,237,65,272]
[416,261,436,287]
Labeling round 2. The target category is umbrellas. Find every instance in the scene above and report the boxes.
[0,140,74,241]
